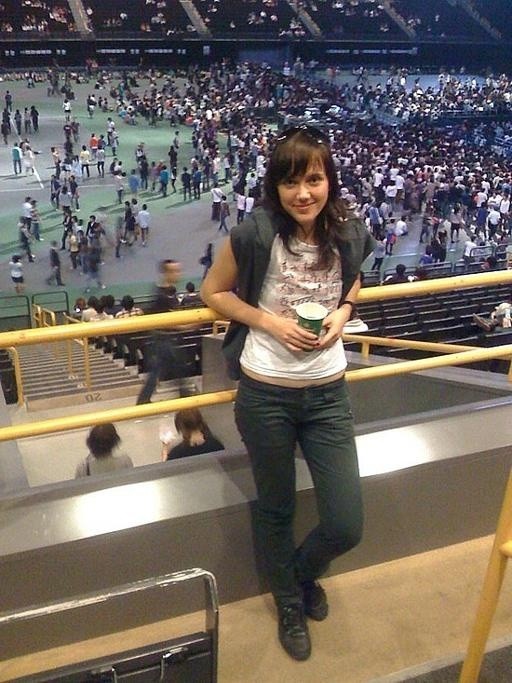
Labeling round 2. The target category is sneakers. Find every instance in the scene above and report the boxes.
[278,581,328,660]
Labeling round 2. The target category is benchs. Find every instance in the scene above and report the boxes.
[0,347,18,404]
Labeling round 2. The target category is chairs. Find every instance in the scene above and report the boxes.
[71,244,512,382]
[0,568,219,683]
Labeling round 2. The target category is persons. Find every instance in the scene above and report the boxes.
[2,0,511,404]
[161,407,224,461]
[74,420,133,476]
[199,127,377,660]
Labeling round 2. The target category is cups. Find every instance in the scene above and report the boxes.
[296,302,325,352]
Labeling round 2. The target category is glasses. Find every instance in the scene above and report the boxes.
[274,124,331,146]
